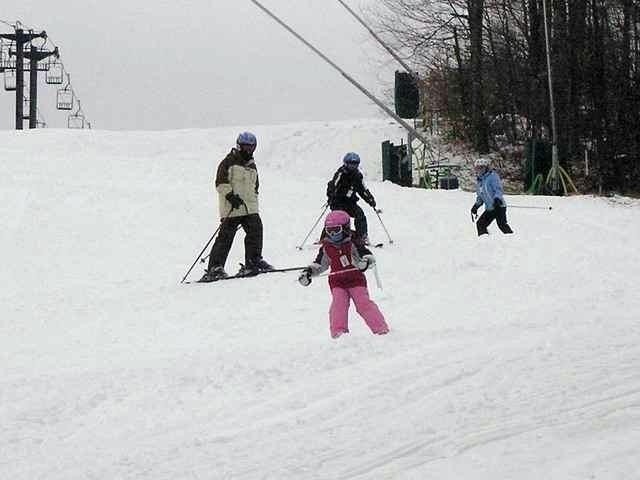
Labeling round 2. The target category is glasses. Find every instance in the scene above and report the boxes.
[326,225,343,235]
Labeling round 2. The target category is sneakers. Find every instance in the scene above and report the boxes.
[207,264,227,278]
[245,260,272,271]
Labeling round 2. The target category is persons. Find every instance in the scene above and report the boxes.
[205,132,274,279]
[470,157,513,237]
[299,210,390,339]
[327,152,380,246]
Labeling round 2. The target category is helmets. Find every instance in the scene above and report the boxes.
[343,153,360,163]
[474,158,488,167]
[326,211,350,226]
[237,133,257,146]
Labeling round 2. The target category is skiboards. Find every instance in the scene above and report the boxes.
[186,259,310,283]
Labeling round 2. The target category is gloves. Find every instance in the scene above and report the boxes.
[299,271,311,286]
[358,256,369,272]
[471,204,478,214]
[494,200,500,208]
[369,200,376,207]
[226,192,243,208]
